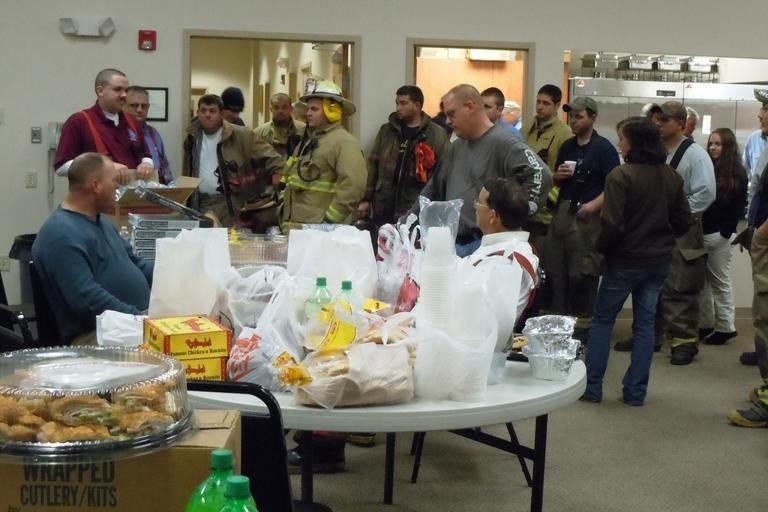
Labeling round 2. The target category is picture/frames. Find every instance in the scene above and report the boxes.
[142,87,169,121]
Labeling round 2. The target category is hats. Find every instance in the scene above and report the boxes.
[563,97,597,114]
[754,88,768,102]
[299,80,356,116]
[222,88,244,111]
[649,101,687,120]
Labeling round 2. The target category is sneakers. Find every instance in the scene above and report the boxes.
[614,328,757,366]
[726,385,768,427]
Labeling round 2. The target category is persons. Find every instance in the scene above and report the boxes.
[740,129,768,366]
[31,151,154,347]
[184,80,573,257]
[466,178,540,330]
[728,88,768,428]
[703,129,748,343]
[542,97,620,316]
[54,69,155,185]
[683,108,700,138]
[123,86,173,183]
[579,117,691,407]
[614,101,716,366]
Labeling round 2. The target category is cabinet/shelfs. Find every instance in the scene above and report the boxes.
[567,52,768,307]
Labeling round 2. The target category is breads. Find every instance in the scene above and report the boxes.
[0,369,189,443]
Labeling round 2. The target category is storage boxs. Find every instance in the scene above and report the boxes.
[0,407,243,512]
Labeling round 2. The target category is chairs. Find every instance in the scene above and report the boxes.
[0,234,63,352]
[185,380,331,512]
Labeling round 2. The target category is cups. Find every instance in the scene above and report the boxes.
[564,160,576,176]
[415,227,494,402]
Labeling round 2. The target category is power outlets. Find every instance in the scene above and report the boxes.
[26,172,37,188]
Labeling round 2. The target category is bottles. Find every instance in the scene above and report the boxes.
[184,447,258,512]
[302,277,333,319]
[218,474,259,512]
[334,280,361,310]
